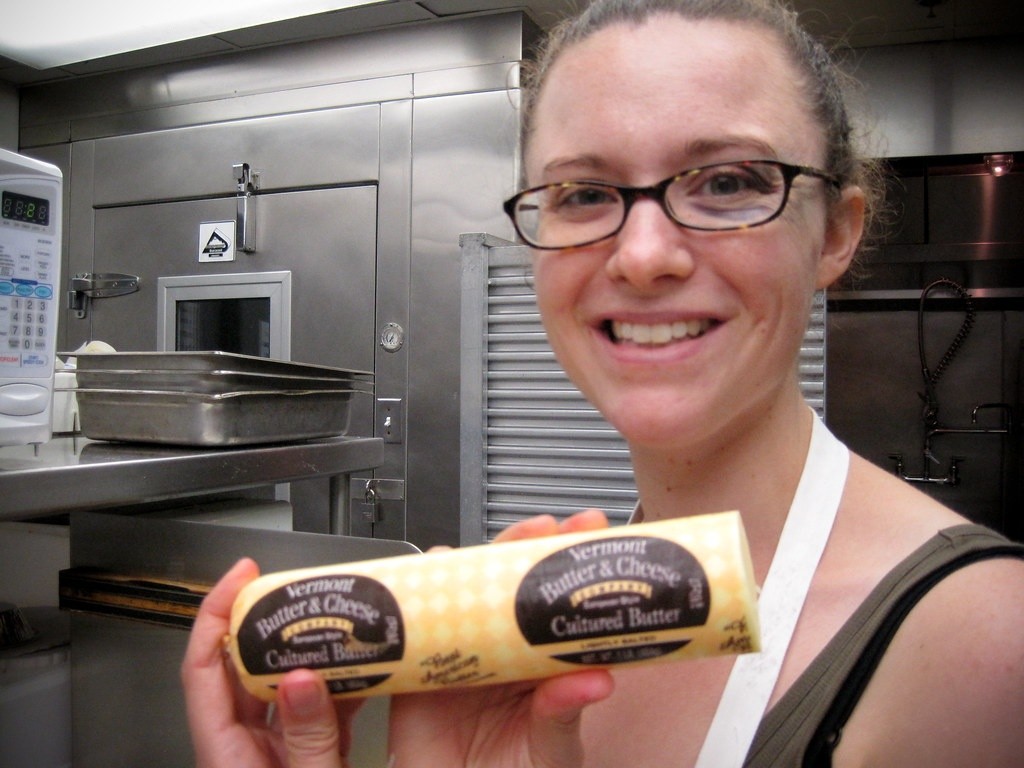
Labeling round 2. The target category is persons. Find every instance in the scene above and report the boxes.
[181,0,1024,768]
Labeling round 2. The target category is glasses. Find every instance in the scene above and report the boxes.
[502,159,842,250]
